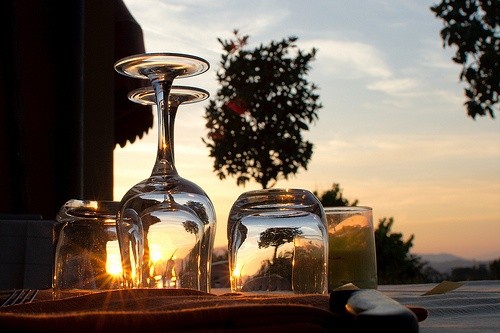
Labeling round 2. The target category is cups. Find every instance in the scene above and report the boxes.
[52,198,128,290]
[321,206,378,287]
[227,190,328,295]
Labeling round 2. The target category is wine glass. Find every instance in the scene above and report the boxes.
[113,51,217,293]
[128,85,210,290]
